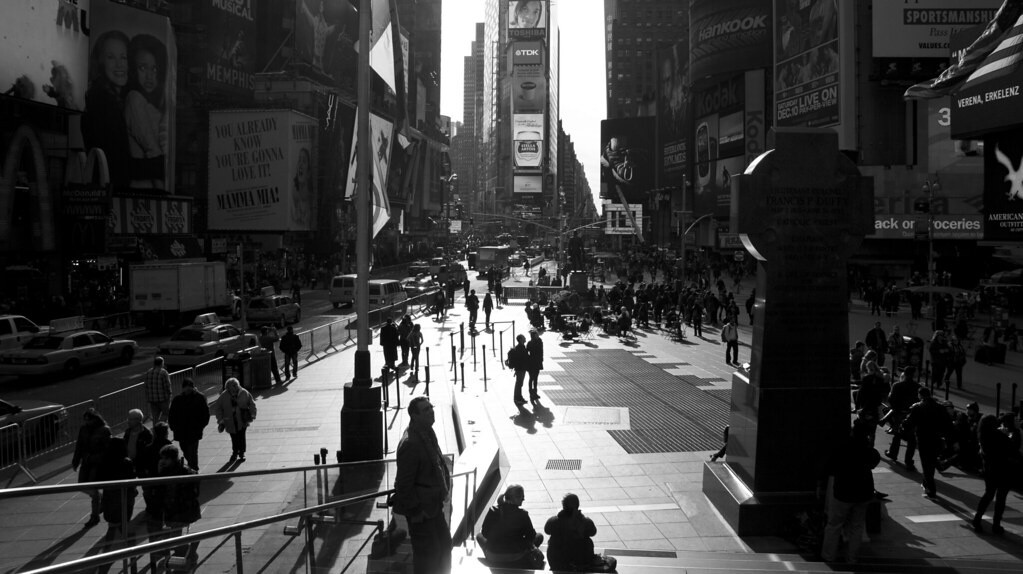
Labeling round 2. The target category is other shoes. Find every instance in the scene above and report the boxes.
[84,493,103,528]
[230,450,244,461]
[530,390,540,399]
[515,396,528,404]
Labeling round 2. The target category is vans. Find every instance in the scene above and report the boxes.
[468,252,477,269]
[329,274,358,309]
[355,279,407,317]
[430,258,451,279]
[438,263,467,287]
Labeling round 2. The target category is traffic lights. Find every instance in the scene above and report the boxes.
[470,218,474,228]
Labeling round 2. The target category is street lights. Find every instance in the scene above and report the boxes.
[921,180,940,319]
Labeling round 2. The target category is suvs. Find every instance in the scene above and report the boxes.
[245,287,301,328]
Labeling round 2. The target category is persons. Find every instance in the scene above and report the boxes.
[291,150,315,231]
[72,231,1023,573]
[78,30,132,187]
[125,34,168,188]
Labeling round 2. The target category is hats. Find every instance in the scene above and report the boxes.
[161,446,178,459]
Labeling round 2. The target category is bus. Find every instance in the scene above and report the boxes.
[516,236,528,250]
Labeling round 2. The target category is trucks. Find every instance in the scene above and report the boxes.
[475,246,511,276]
[129,261,241,328]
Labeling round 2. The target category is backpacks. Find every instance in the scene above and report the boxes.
[505,348,516,369]
[183,466,200,497]
[721,325,730,343]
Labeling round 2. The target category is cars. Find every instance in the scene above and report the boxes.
[524,239,551,257]
[409,262,429,276]
[154,313,257,366]
[398,273,436,297]
[0,315,56,347]
[514,251,527,261]
[509,254,522,266]
[0,399,68,459]
[0,315,138,380]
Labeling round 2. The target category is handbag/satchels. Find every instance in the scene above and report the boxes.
[592,552,617,573]
[241,408,253,424]
